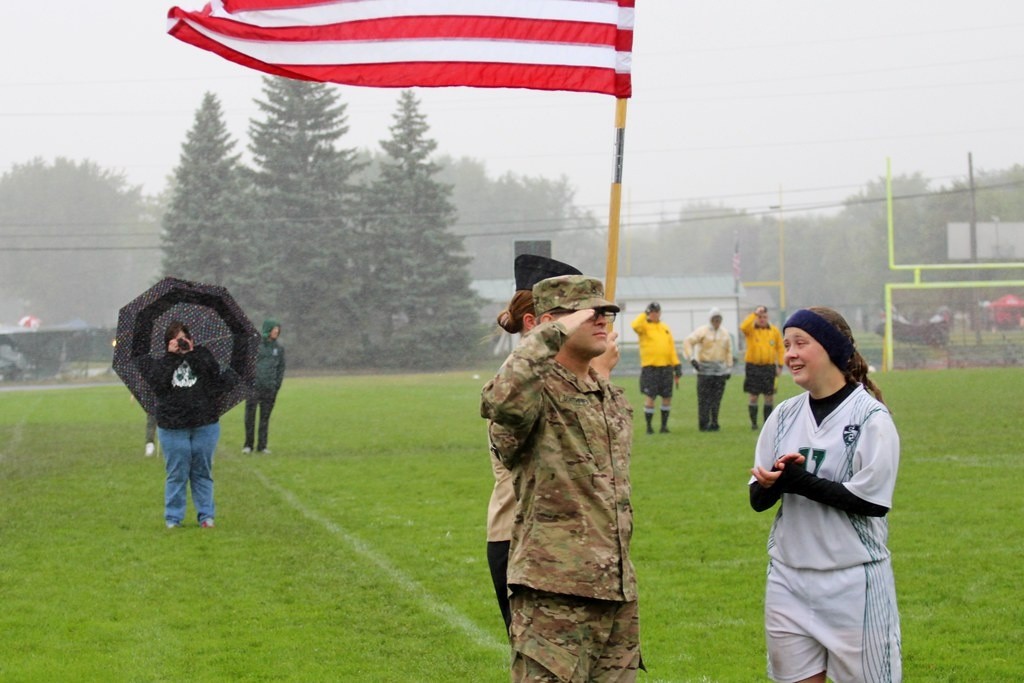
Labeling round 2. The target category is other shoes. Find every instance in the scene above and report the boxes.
[752,423,758,430]
[659,427,669,433]
[646,427,654,435]
[699,425,707,430]
[242,446,251,455]
[144,442,155,457]
[707,423,719,432]
[260,448,270,455]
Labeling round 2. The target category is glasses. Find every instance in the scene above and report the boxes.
[547,309,617,325]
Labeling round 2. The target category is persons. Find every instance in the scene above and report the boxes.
[749,306,904,683]
[479,254,646,683]
[240,319,287,454]
[683,306,733,431]
[144,414,156,457]
[632,302,683,433]
[739,305,786,432]
[148,321,220,531]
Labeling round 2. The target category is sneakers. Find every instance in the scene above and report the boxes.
[166,519,181,528]
[197,511,217,530]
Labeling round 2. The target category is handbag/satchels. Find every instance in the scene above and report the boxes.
[203,370,236,403]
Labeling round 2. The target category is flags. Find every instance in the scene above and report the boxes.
[160,0,635,103]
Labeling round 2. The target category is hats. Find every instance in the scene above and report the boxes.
[511,253,584,291]
[646,303,660,312]
[709,308,723,321]
[531,274,621,316]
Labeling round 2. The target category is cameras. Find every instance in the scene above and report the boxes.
[176,339,190,351]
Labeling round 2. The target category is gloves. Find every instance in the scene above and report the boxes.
[674,365,683,379]
[691,359,700,372]
[726,367,731,379]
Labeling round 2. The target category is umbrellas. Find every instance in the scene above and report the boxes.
[112,276,262,423]
